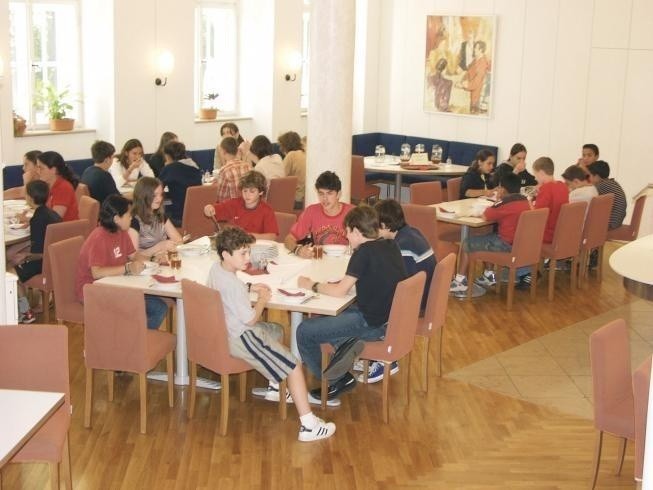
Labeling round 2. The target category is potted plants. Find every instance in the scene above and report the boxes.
[200,93,220,120]
[46,85,75,130]
[13,110,26,137]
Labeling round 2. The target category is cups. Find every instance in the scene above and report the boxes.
[375,144,385,162]
[314,246,323,259]
[168,250,181,271]
[400,143,410,161]
[415,143,425,153]
[431,143,442,164]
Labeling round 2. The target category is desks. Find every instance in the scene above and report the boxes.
[608,234,653,301]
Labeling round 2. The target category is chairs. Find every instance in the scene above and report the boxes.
[598,185,652,281]
[627,357,652,490]
[579,194,612,288]
[590,318,632,489]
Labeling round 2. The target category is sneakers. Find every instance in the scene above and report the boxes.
[311,372,354,402]
[264,380,292,404]
[449,276,468,292]
[36,293,53,312]
[19,309,36,323]
[298,418,336,441]
[475,273,496,287]
[356,359,398,384]
[516,272,541,288]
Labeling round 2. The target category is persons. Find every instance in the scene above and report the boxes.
[431,25,489,117]
[2,123,307,324]
[448,140,627,294]
[285,170,356,260]
[353,197,437,384]
[205,224,336,443]
[295,203,409,402]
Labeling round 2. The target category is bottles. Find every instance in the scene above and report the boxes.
[205,169,210,183]
[446,156,452,166]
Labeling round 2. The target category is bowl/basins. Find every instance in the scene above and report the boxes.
[181,248,201,257]
[323,244,347,256]
[138,261,159,275]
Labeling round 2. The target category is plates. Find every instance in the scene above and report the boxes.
[254,239,279,259]
